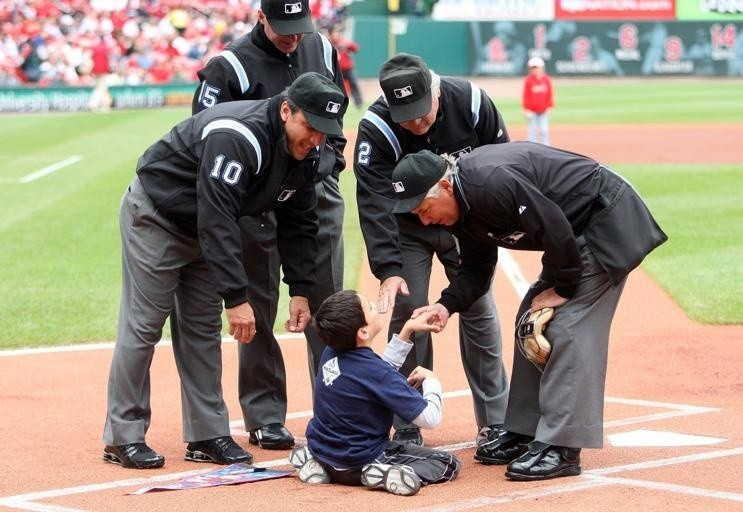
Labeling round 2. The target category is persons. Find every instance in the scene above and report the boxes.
[0,0,363,107]
[289,289,461,495]
[523,57,555,148]
[191,0,350,449]
[102,71,346,470]
[391,140,668,481]
[353,53,511,447]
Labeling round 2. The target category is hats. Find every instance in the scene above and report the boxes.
[528,57,546,68]
[378,53,433,124]
[261,0,314,36]
[389,149,448,214]
[288,72,346,136]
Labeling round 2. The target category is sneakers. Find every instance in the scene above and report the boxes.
[359,461,424,497]
[102,438,165,470]
[183,434,254,467]
[249,422,295,450]
[477,425,508,444]
[391,427,425,450]
[289,445,331,485]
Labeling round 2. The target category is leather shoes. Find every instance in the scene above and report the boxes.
[504,445,582,481]
[473,431,535,465]
